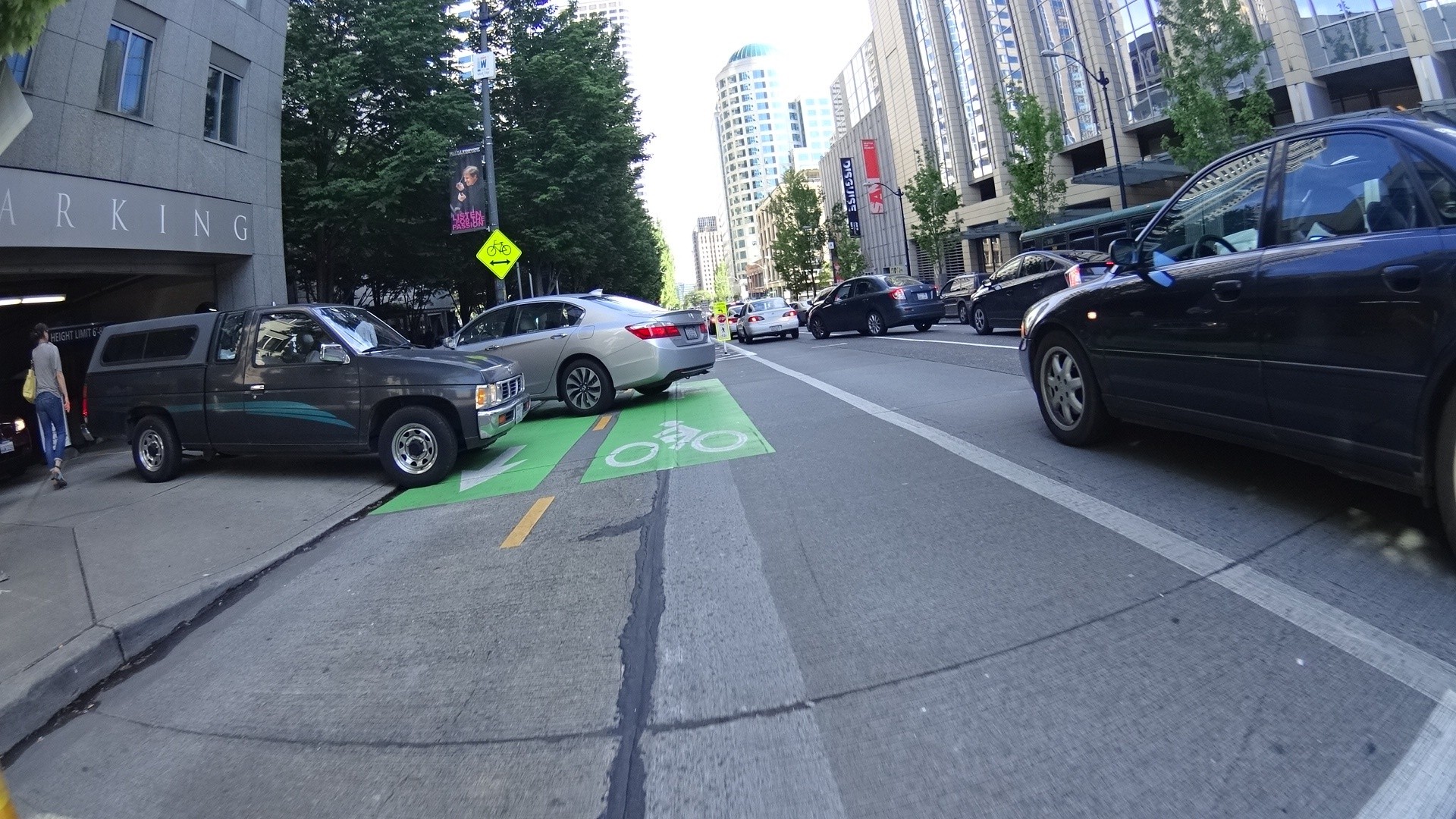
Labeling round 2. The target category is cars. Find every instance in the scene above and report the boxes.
[969,250,1111,336]
[788,301,813,326]
[805,281,851,327]
[432,289,718,417]
[733,297,800,344]
[0,407,37,476]
[806,274,946,340]
[702,301,745,339]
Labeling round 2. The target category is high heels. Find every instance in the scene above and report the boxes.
[51,478,63,490]
[53,466,67,486]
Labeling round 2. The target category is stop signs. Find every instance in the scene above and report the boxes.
[718,315,727,324]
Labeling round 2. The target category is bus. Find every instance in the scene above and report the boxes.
[1019,199,1173,261]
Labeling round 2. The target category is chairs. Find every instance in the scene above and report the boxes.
[520,315,537,333]
[1295,186,1365,243]
[545,310,570,329]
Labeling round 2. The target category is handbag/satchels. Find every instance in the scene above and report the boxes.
[22,358,37,403]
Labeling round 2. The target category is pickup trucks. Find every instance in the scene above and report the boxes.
[78,300,531,489]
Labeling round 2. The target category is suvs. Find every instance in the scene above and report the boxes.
[935,271,994,324]
[1017,90,1455,560]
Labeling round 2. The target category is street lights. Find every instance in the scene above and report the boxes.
[803,225,837,283]
[860,182,912,277]
[727,275,745,300]
[1040,48,1131,210]
[749,262,770,298]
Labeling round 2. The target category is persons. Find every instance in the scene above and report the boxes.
[354,315,377,351]
[31,323,71,490]
[304,327,333,363]
[455,164,484,219]
